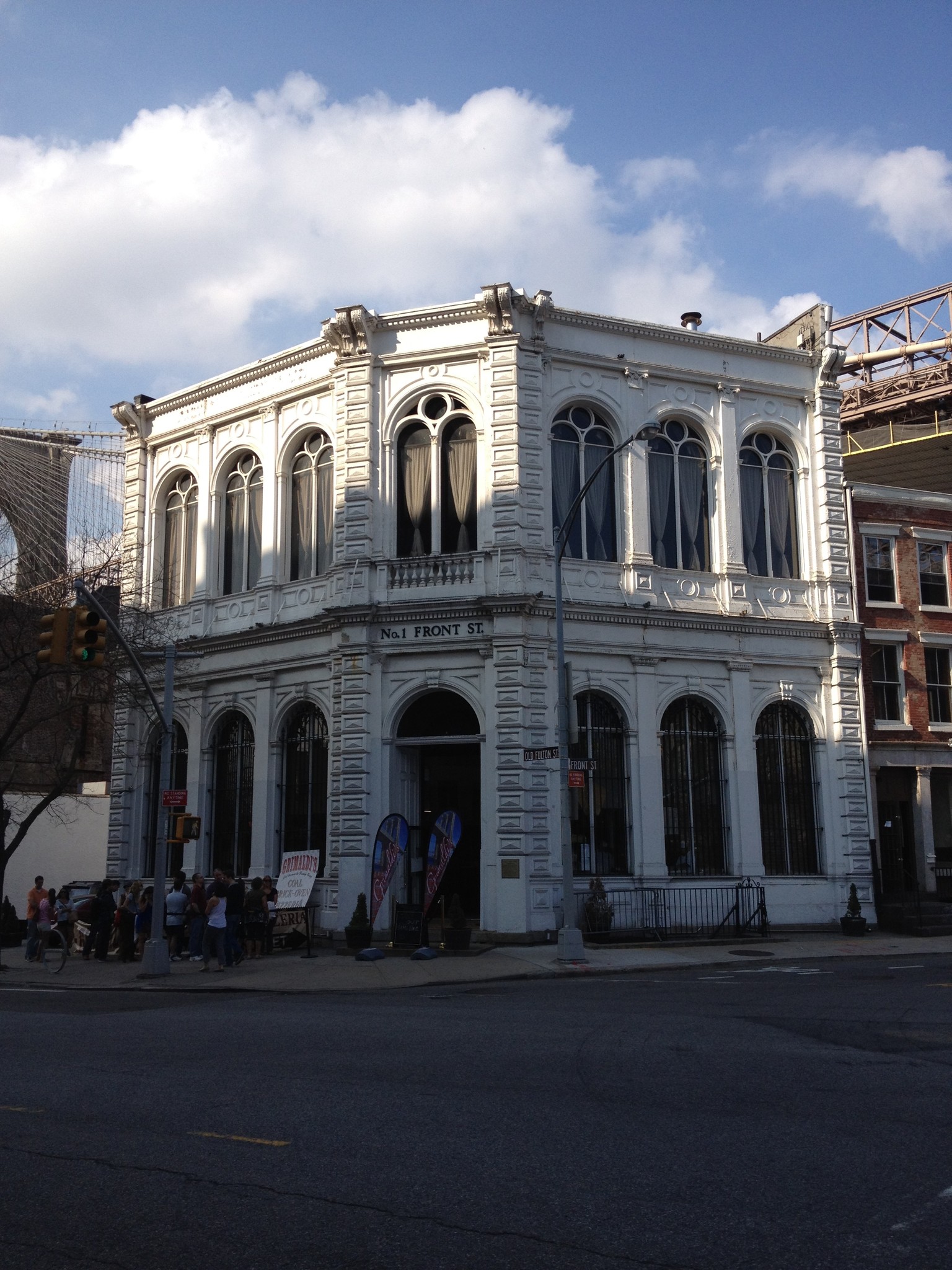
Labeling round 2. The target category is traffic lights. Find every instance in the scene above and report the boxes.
[35,607,72,666]
[175,816,202,841]
[70,605,109,669]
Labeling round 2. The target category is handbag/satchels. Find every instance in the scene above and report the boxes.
[113,910,121,927]
[32,905,39,921]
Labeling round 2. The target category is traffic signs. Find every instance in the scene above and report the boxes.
[162,789,189,806]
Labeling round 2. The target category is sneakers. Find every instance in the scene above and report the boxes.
[199,955,203,958]
[189,956,202,961]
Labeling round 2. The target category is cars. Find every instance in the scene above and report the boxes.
[43,899,94,948]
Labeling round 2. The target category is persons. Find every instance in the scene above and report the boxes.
[205,869,227,957]
[54,888,74,949]
[25,875,48,962]
[166,880,187,962]
[243,877,270,959]
[260,875,278,955]
[82,880,121,960]
[222,869,244,968]
[199,884,228,972]
[121,880,143,963]
[76,900,94,947]
[169,871,191,950]
[35,889,56,962]
[96,879,117,963]
[188,873,209,961]
[134,886,154,962]
[117,881,133,960]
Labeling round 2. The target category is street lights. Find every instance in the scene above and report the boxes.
[555,419,664,965]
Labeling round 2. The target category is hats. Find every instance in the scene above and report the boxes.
[123,882,132,888]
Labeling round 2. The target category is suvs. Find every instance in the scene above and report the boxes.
[55,881,103,900]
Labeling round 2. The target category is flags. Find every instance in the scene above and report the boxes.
[423,812,462,921]
[370,813,410,928]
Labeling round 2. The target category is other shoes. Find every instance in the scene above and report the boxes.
[199,966,209,971]
[67,949,71,956]
[40,958,50,962]
[170,955,183,961]
[25,957,32,961]
[223,963,233,968]
[235,952,244,965]
[134,952,141,955]
[214,968,224,972]
[36,957,40,960]
[83,953,89,960]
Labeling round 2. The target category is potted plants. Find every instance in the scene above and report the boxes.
[345,892,373,949]
[840,883,866,937]
[581,879,614,942]
